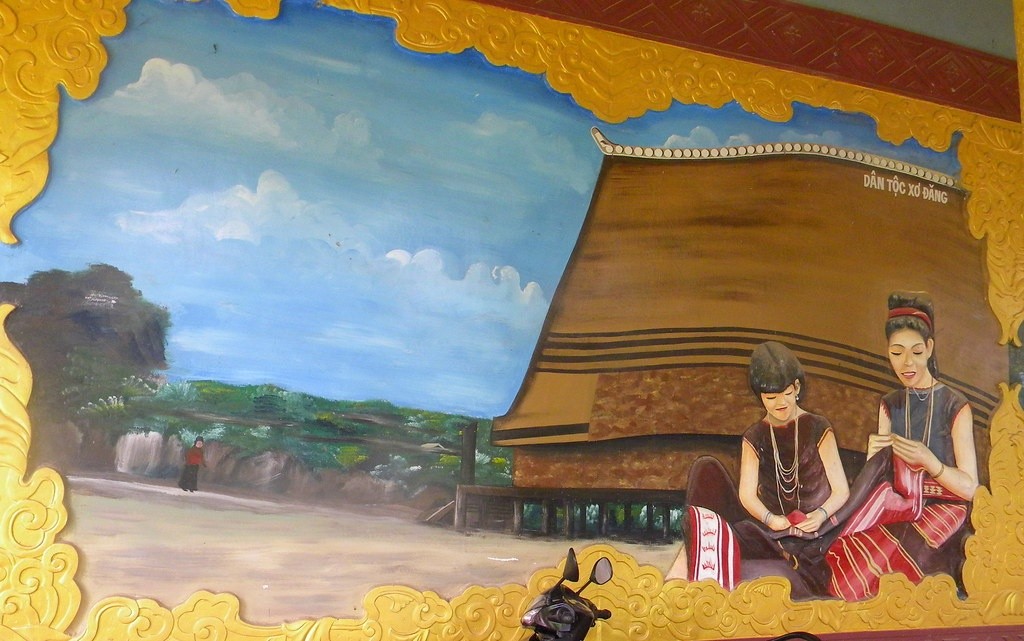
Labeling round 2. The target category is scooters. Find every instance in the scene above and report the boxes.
[522,548,820,641]
